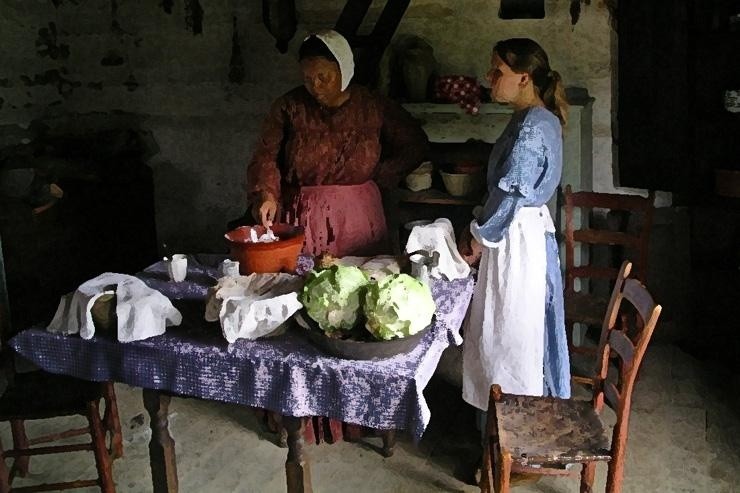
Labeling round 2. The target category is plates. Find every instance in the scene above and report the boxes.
[405,218,433,231]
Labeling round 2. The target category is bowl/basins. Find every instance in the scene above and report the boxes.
[438,165,484,197]
[293,310,439,361]
[222,222,305,275]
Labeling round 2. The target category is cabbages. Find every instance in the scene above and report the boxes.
[295,261,368,337]
[365,271,437,344]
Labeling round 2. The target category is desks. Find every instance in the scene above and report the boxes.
[8,253,478,493]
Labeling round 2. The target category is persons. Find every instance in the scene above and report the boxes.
[456,38,572,486]
[246,30,380,230]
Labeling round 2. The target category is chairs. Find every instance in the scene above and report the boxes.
[480,261,663,493]
[561,184,654,405]
[0,306,125,492]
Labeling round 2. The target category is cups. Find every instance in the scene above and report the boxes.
[163,254,188,283]
[410,253,434,283]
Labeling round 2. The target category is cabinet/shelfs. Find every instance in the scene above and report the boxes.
[380,100,581,260]
